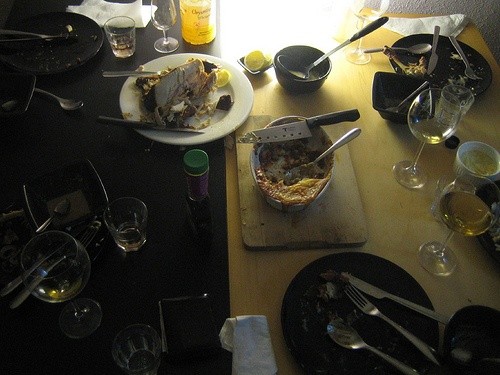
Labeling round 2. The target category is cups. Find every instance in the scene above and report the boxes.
[430,174,475,222]
[433,85,475,134]
[102,16,166,375]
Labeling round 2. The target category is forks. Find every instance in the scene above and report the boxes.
[343,282,446,368]
[448,36,483,80]
[9,226,98,309]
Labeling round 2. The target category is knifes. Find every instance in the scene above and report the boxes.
[234,107,359,144]
[341,271,450,325]
[97,113,204,134]
[428,26,440,74]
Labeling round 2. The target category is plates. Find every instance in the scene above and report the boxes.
[0,12,273,228]
[238,33,500,374]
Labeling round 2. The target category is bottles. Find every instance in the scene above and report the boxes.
[181,1,217,253]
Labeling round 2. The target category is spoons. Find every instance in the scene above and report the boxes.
[284,128,361,183]
[34,87,83,110]
[327,321,421,375]
[364,44,432,54]
[450,348,500,366]
[385,80,430,113]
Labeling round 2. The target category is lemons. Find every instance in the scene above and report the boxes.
[244,49,267,72]
[216,69,230,87]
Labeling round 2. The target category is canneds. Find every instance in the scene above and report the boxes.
[184,150,209,202]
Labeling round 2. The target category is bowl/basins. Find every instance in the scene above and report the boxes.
[454,141,500,186]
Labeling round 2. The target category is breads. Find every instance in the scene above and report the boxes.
[254,168,331,205]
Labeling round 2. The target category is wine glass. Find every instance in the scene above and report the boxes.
[19,230,101,340]
[151,0,180,54]
[392,86,461,191]
[416,173,500,277]
[343,0,390,65]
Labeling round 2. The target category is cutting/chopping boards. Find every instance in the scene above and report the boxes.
[235,122,368,252]
